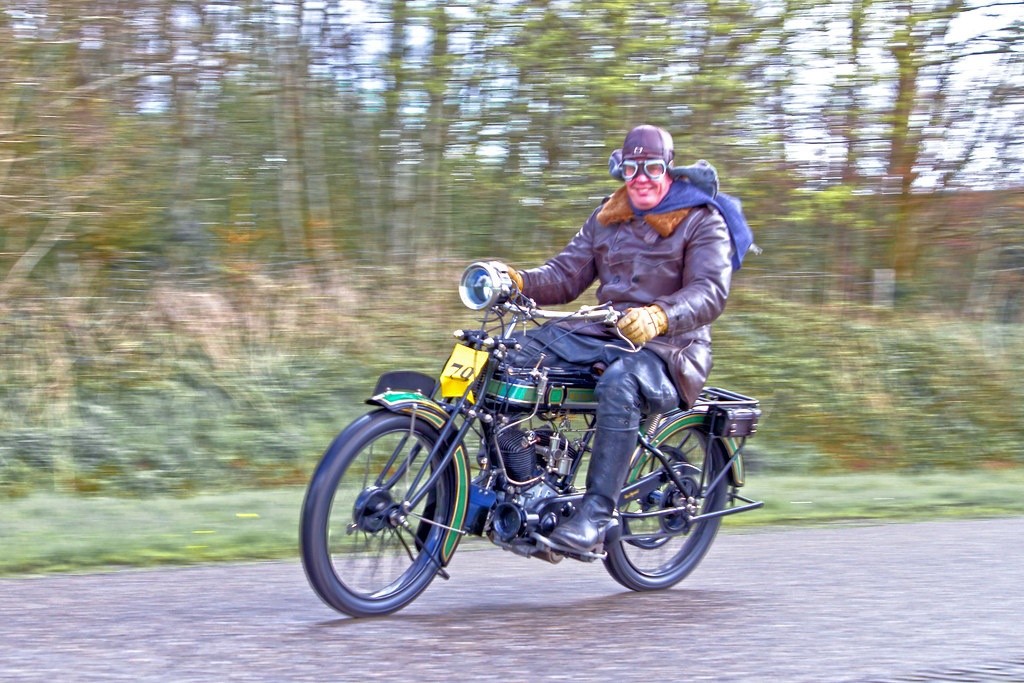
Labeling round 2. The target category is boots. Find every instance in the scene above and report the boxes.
[547,424,638,554]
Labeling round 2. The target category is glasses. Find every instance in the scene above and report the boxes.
[620,158,674,181]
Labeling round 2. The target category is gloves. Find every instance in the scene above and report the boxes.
[617,303,669,345]
[505,263,523,302]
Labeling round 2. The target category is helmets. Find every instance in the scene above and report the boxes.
[621,125,675,165]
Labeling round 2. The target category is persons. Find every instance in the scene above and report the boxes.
[469,126,732,552]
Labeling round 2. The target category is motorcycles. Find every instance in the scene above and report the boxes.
[298,260,761,618]
